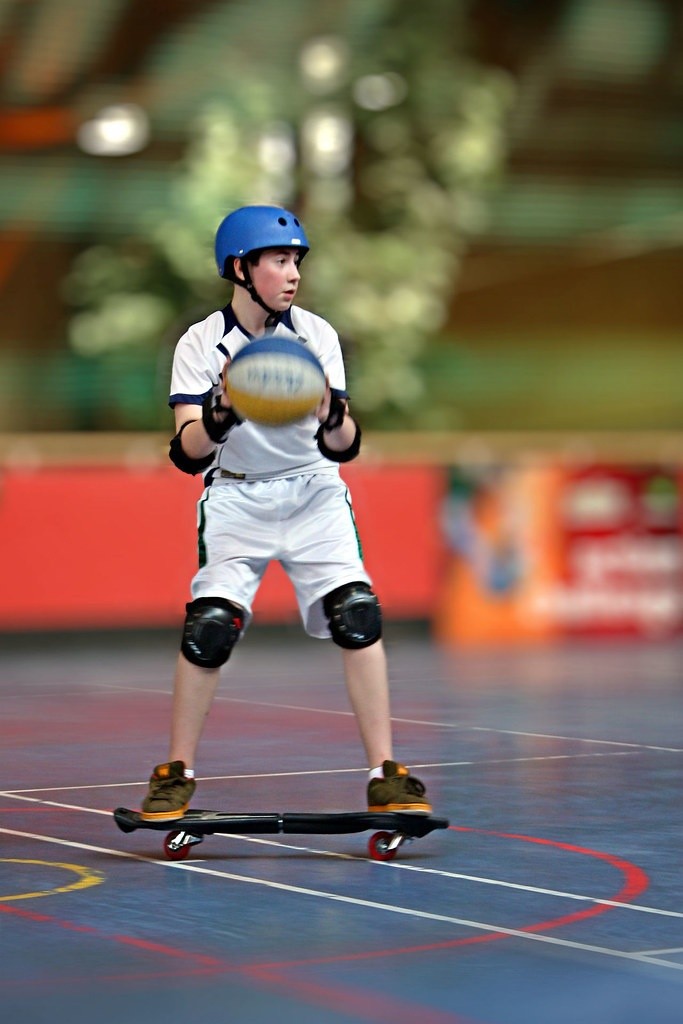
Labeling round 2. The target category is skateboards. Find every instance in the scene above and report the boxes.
[112,805,451,862]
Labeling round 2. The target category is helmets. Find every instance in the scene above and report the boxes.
[215,204,309,280]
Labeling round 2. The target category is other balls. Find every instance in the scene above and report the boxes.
[223,334,328,429]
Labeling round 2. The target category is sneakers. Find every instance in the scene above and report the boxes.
[139,760,197,823]
[366,759,432,816]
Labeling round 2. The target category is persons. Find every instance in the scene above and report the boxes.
[140,205,431,821]
